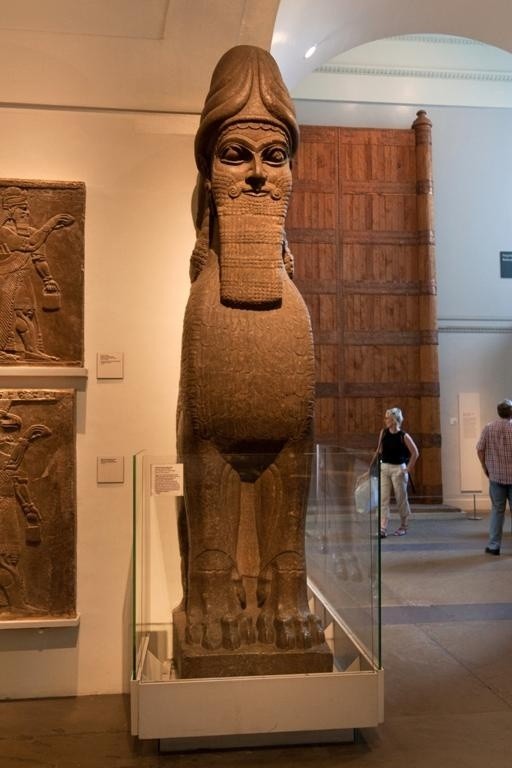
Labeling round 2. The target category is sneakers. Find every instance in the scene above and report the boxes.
[485,547,499,555]
[376,531,387,537]
[394,528,406,536]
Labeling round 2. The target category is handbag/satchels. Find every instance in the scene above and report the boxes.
[370,453,380,478]
[355,477,379,513]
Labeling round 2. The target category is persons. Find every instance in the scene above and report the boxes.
[475,399,512,555]
[362,407,420,538]
